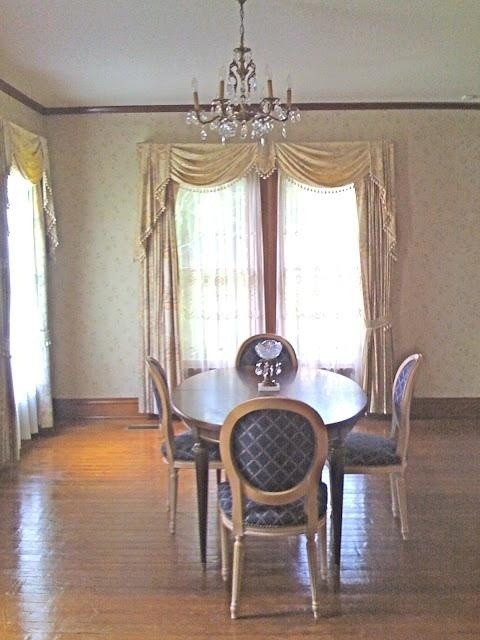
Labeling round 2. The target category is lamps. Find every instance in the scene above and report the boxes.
[185,0,302,146]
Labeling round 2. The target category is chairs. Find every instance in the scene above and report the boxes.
[143,357,225,534]
[235,334,298,367]
[320,354,424,541]
[216,397,328,619]
